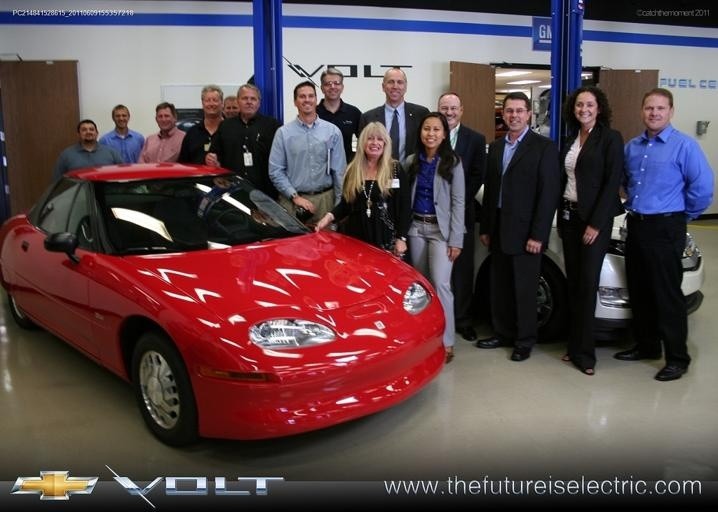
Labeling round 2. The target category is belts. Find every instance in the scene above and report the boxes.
[300,186,333,195]
[415,216,437,224]
[628,210,681,221]
[562,200,578,210]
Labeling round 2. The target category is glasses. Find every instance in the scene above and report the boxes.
[324,80,342,85]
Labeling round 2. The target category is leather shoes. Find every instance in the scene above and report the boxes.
[614,348,661,360]
[655,366,686,380]
[446,328,531,363]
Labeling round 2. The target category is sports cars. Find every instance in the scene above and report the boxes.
[0,160,448,449]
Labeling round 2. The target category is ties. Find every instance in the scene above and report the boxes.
[390,110,399,160]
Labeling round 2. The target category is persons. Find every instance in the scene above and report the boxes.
[362,67,430,164]
[176,86,231,166]
[220,93,241,120]
[207,84,282,203]
[615,88,714,380]
[435,93,486,346]
[138,103,187,165]
[52,120,123,181]
[97,105,144,168]
[243,203,289,233]
[402,113,465,365]
[207,176,247,194]
[478,90,561,362]
[267,83,349,237]
[559,86,627,375]
[314,120,413,275]
[310,68,362,164]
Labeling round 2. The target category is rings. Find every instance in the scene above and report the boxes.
[588,237,592,239]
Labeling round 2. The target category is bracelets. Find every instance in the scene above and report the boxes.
[397,234,408,243]
[324,209,338,222]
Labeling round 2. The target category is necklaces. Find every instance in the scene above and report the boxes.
[356,160,384,219]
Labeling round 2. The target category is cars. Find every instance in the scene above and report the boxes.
[420,142,703,349]
